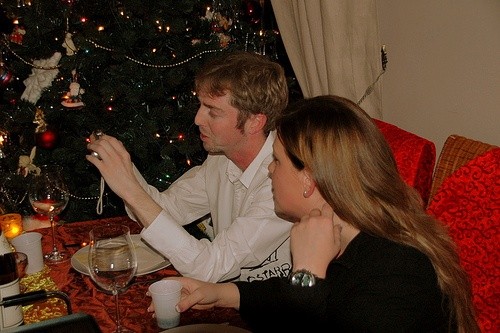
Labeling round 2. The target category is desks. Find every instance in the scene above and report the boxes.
[19,216,243,333]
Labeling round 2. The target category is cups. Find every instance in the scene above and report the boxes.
[10,232,45,275]
[4,252,28,281]
[148,280,185,329]
[0,213,23,239]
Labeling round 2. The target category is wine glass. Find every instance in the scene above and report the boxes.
[29,163,72,265]
[88,224,138,333]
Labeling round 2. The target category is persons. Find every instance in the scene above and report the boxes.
[86,51,295,282]
[146,95,482,333]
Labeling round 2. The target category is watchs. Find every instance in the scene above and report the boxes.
[290,269,323,287]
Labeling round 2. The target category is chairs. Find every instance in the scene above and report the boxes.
[370,116,500,331]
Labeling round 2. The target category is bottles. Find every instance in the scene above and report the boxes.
[0,227,23,331]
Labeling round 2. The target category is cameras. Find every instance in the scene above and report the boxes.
[91,130,102,160]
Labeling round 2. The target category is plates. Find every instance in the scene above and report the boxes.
[70,234,175,276]
